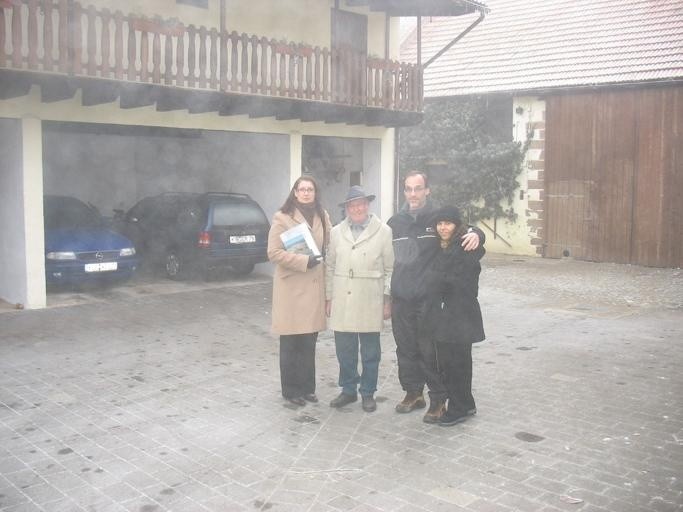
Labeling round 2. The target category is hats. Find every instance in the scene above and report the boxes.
[432,205,463,228]
[336,185,376,208]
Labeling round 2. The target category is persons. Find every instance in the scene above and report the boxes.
[385,170,487,424]
[424,204,485,426]
[324,185,392,412]
[266,174,332,406]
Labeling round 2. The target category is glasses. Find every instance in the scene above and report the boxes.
[295,187,315,193]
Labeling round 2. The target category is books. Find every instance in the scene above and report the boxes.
[279,221,323,261]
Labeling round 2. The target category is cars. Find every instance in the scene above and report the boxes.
[42,194,140,288]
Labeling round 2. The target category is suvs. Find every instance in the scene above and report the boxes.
[108,193,270,281]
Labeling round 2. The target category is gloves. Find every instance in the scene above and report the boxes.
[307,255,322,268]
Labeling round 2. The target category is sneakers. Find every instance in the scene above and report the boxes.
[422,399,446,423]
[361,394,377,412]
[395,392,425,413]
[436,404,479,427]
[302,392,319,402]
[329,392,357,407]
[285,395,305,407]
